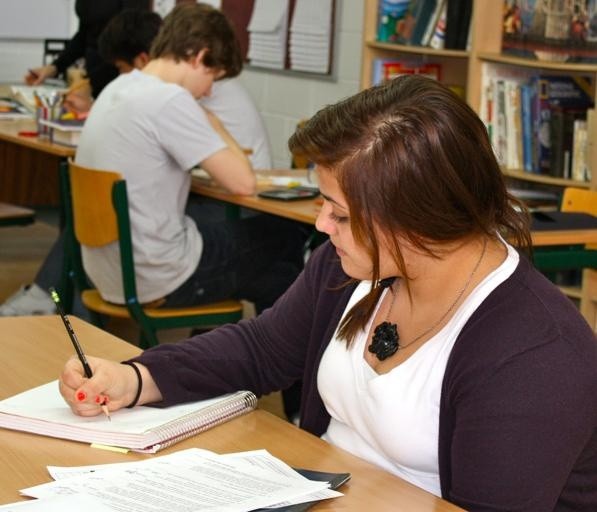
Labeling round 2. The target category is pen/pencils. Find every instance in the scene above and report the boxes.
[32,90,63,134]
[49,286,112,419]
[28,70,37,78]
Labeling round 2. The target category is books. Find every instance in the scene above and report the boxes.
[481,63,596,181]
[502,2,597,63]
[373,0,476,52]
[38,118,83,147]
[372,60,443,87]
[0,373,258,455]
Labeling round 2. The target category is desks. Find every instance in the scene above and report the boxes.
[0,313,466,512]
[0,82,597,332]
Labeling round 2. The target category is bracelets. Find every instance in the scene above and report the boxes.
[121,360,143,408]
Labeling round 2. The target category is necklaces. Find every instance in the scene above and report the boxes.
[367,235,487,362]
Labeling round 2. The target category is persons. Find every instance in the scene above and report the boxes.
[25,0,152,102]
[0,9,272,316]
[58,74,597,512]
[72,3,302,422]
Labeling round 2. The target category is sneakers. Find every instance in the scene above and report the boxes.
[0,281,57,316]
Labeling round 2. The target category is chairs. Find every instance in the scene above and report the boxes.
[57,154,245,353]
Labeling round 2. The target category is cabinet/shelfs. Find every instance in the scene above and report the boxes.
[356,0,595,200]
